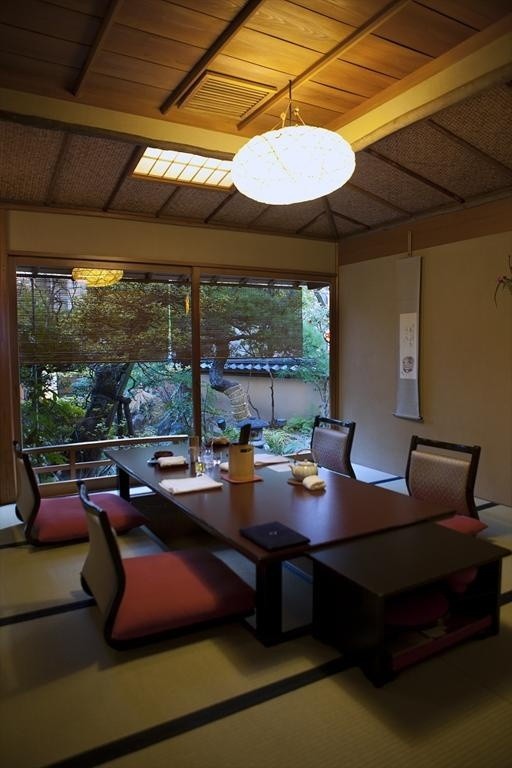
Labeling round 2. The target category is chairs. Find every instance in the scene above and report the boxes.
[72,478,256,652]
[310,415,356,482]
[12,440,150,549]
[406,435,481,522]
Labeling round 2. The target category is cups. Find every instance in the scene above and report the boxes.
[195,461,206,476]
[201,446,214,469]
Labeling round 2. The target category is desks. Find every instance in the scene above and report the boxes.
[102,442,457,648]
[305,519,512,689]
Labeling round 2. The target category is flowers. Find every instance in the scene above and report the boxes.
[493,254,512,309]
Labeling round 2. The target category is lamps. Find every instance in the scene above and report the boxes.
[72,267,123,289]
[180,274,191,315]
[230,79,356,206]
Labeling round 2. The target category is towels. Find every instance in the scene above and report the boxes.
[157,456,186,468]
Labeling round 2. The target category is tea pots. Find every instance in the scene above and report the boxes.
[288,449,319,482]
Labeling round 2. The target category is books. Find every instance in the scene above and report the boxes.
[240,518,311,550]
[253,452,290,464]
